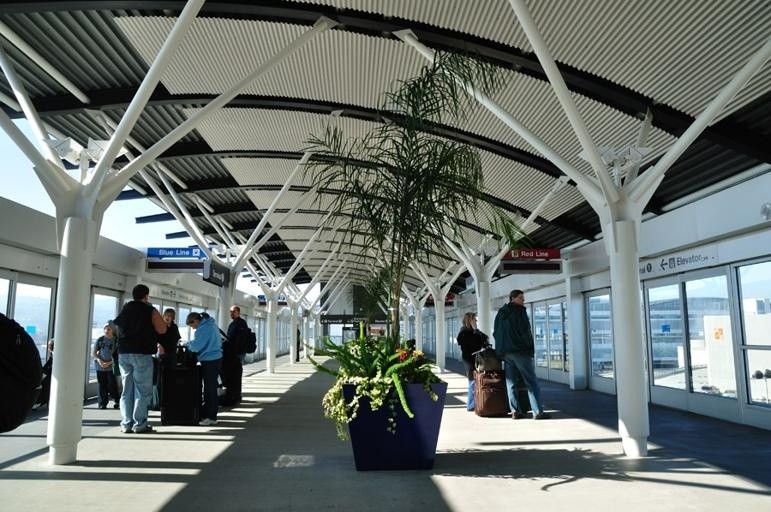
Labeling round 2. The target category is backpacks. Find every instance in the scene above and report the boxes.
[233,318,257,354]
[218,327,236,366]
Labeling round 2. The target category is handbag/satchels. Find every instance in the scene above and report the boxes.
[169,345,198,366]
[111,347,122,376]
[474,348,502,373]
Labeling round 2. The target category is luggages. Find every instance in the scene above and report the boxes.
[160,363,203,427]
[472,369,509,417]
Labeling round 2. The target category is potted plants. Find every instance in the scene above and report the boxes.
[299,50,544,470]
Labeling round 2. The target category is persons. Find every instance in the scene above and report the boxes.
[219,304,248,407]
[112,284,167,435]
[155,307,181,357]
[456,312,490,412]
[91,325,120,410]
[493,289,552,420]
[182,312,224,426]
[32,338,55,411]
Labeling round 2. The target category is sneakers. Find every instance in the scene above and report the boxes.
[198,418,218,426]
[467,407,475,412]
[134,424,152,433]
[532,413,553,420]
[511,412,531,419]
[119,425,132,433]
[98,404,120,409]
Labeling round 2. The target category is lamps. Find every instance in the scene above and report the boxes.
[209,243,246,266]
[578,144,655,189]
[42,136,128,184]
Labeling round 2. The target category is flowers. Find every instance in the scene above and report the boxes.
[311,321,446,443]
